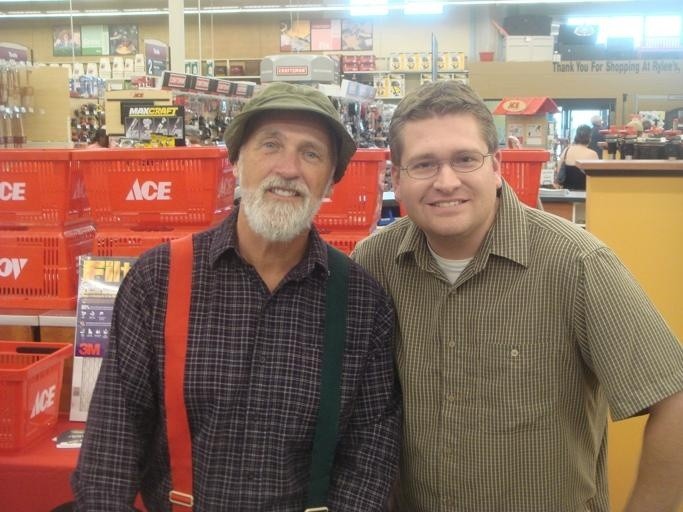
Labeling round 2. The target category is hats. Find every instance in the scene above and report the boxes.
[222,80,360,185]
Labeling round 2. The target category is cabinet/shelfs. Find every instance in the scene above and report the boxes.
[0,55,471,153]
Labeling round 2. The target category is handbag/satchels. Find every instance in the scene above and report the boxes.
[555,161,586,191]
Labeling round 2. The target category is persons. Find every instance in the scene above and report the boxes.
[68,82,404,512]
[87,114,109,148]
[552,109,657,189]
[348,80,683,512]
[113,34,136,55]
[53,29,80,57]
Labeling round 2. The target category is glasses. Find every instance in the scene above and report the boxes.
[397,148,496,181]
